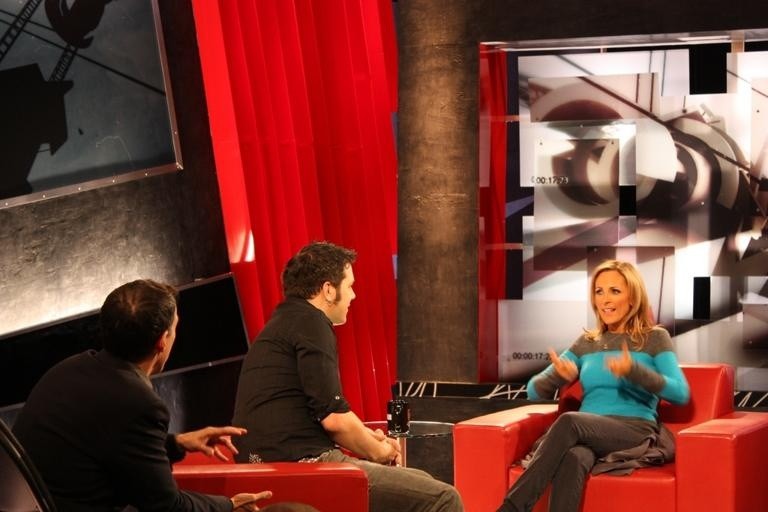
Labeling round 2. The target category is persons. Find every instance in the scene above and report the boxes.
[495,260,691,509]
[10,276,272,510]
[221,241,460,511]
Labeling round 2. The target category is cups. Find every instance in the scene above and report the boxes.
[387,399,410,434]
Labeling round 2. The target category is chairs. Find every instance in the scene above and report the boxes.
[171,439,370,512]
[0,421,58,511]
[452,361,768,512]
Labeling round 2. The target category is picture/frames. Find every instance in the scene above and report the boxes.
[1,1,184,213]
